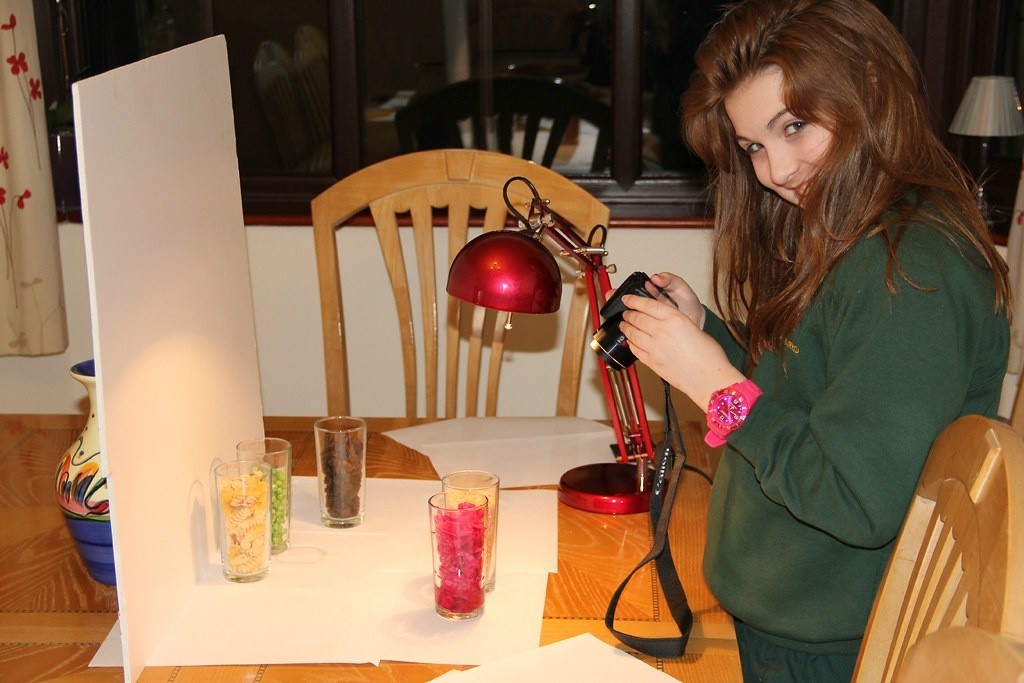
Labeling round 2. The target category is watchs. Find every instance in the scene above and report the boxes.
[702,379,763,448]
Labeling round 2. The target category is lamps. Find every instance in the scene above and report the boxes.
[947,76,1024,228]
[445,175,670,516]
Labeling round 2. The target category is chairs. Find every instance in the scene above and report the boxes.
[392,64,626,178]
[850,414,1024,683]
[310,149,609,416]
[252,22,332,177]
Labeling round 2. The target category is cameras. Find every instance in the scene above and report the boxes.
[590,271,679,371]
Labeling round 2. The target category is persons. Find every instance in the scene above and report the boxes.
[604,0,1016,682]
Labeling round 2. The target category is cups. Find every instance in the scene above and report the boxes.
[212,460,272,583]
[428,491,489,621]
[236,436,293,555]
[440,469,500,594]
[313,415,368,528]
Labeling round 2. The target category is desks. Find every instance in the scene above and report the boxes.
[0,412,745,683]
